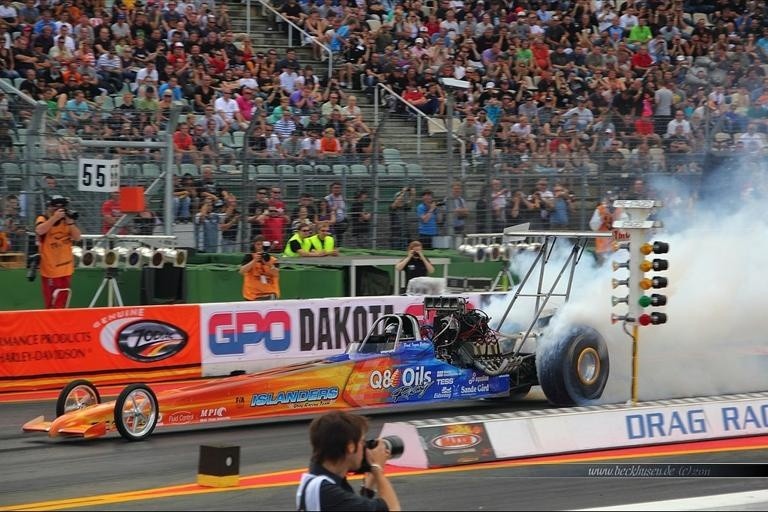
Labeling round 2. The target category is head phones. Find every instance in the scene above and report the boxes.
[250,237,256,251]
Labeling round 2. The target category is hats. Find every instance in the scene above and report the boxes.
[44,195,71,207]
[0,0,768,176]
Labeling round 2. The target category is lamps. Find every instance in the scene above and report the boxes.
[459,234,547,263]
[69,235,188,271]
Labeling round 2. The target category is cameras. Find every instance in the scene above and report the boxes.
[413,252,419,257]
[406,189,411,191]
[260,241,271,265]
[25,254,41,281]
[435,202,446,206]
[60,207,78,220]
[355,435,403,474]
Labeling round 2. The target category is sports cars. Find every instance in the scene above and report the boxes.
[19,229,621,444]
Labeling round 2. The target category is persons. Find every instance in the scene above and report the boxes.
[295,409,402,511]
[375,322,404,353]
[0,1,768,308]
